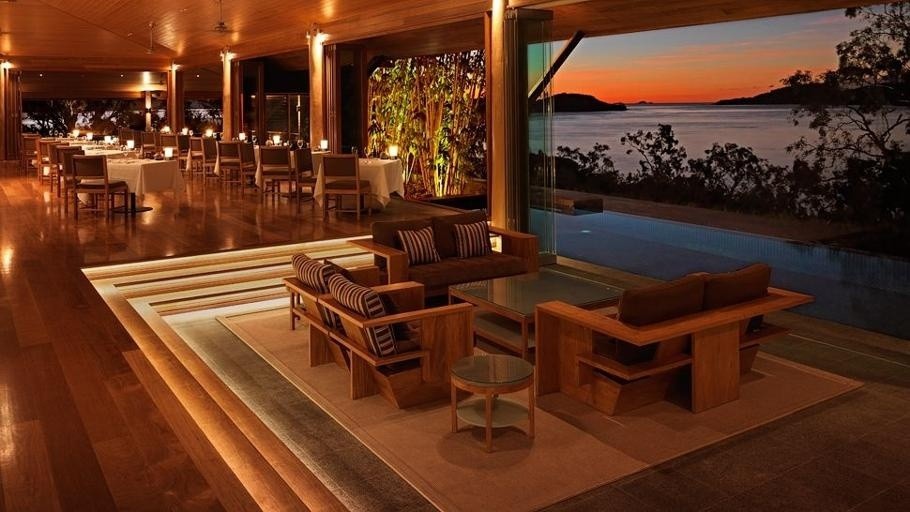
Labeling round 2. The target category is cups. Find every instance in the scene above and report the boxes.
[67,133,70,138]
[58,133,63,138]
[82,136,86,140]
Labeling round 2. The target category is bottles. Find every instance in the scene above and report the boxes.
[120,145,123,151]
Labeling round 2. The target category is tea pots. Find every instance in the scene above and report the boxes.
[380,151,388,159]
[151,151,164,160]
[123,144,129,151]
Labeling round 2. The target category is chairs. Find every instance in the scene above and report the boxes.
[63,151,94,212]
[22,136,40,172]
[201,137,227,186]
[154,133,166,149]
[38,140,61,184]
[134,130,144,148]
[258,145,296,204]
[237,142,259,198]
[48,143,69,193]
[295,149,318,212]
[141,132,156,158]
[159,134,176,154]
[120,128,135,145]
[57,146,82,198]
[188,138,203,180]
[72,155,128,225]
[176,134,191,161]
[215,141,241,191]
[320,153,372,220]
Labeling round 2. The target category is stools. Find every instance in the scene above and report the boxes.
[449,353,534,453]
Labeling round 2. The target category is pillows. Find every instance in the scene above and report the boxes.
[454,220,491,256]
[614,274,704,359]
[689,262,771,310]
[329,273,396,355]
[291,253,336,294]
[397,227,441,265]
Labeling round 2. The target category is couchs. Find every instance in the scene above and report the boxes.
[534,273,816,415]
[346,210,538,298]
[283,265,474,408]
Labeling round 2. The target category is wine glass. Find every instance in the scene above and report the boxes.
[150,127,153,132]
[297,140,303,149]
[74,137,78,142]
[189,130,193,138]
[351,146,358,155]
[134,148,140,163]
[364,147,370,163]
[49,131,53,136]
[123,148,129,162]
[145,127,148,132]
[54,130,58,136]
[244,132,248,143]
[252,130,256,147]
[92,140,97,151]
[213,133,217,139]
